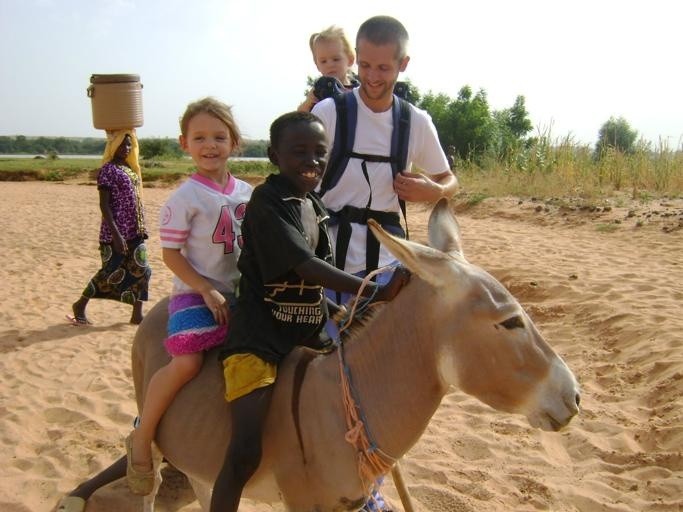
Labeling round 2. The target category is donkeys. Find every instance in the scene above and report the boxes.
[50,196,581,512]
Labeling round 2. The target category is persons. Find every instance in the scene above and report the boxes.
[309,15,459,306]
[71,129,152,325]
[297,23,361,115]
[210,112,410,512]
[126,96,256,495]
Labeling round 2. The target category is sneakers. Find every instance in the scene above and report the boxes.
[125,434,154,496]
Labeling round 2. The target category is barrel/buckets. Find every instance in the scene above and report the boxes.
[86,71,145,132]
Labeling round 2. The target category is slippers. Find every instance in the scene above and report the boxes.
[66,314,92,327]
